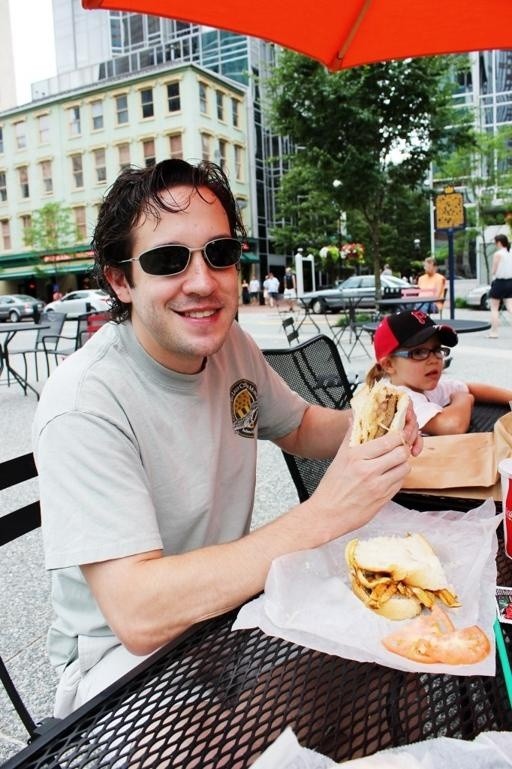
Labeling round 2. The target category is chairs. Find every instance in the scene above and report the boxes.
[0,451,106,743]
[248,332,354,510]
[278,315,359,412]
[5,309,68,397]
[282,289,450,365]
[41,309,113,377]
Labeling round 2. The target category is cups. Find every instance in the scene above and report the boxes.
[496,456,511,560]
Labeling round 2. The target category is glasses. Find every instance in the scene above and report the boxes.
[115,236,243,278]
[391,344,451,362]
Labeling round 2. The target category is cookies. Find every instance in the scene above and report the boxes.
[383,606,489,665]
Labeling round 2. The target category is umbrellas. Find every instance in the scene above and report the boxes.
[77,1,512,77]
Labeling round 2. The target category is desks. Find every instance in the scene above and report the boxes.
[0,321,52,402]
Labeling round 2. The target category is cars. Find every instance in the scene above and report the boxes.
[297,275,418,313]
[0,289,112,323]
[466,284,506,310]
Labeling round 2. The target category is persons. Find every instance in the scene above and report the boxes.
[481,232,512,340]
[32,153,425,768]
[282,266,298,313]
[51,287,63,302]
[242,279,249,304]
[263,272,281,308]
[249,273,260,306]
[381,261,394,277]
[349,306,512,444]
[411,255,445,318]
[263,275,269,304]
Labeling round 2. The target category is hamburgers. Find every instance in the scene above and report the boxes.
[347,378,410,443]
[344,530,462,621]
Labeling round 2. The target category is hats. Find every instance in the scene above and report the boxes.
[372,309,459,360]
